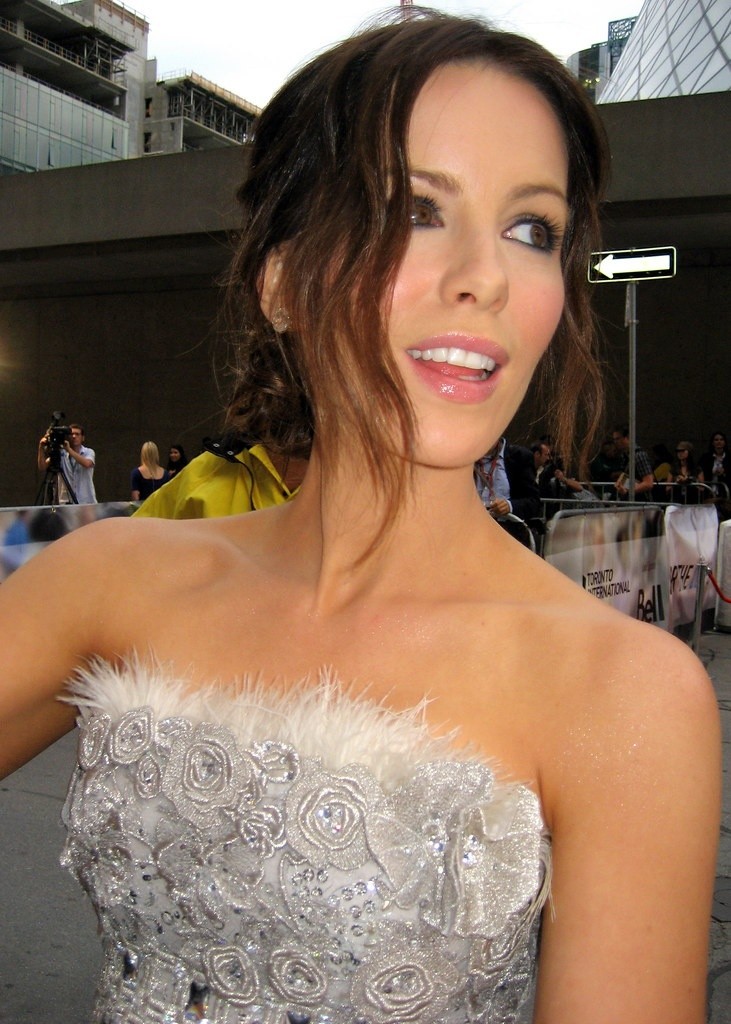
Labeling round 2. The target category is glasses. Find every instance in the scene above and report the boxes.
[71,433,81,437]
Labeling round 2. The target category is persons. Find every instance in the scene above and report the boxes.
[474,440,544,559]
[38,425,99,504]
[129,430,309,519]
[530,427,731,510]
[166,446,189,476]
[0,6,722,1024]
[131,442,169,501]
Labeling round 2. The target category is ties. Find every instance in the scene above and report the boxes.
[480,442,502,501]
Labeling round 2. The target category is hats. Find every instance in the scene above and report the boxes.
[676,442,694,452]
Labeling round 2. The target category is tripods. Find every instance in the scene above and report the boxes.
[33,443,80,505]
